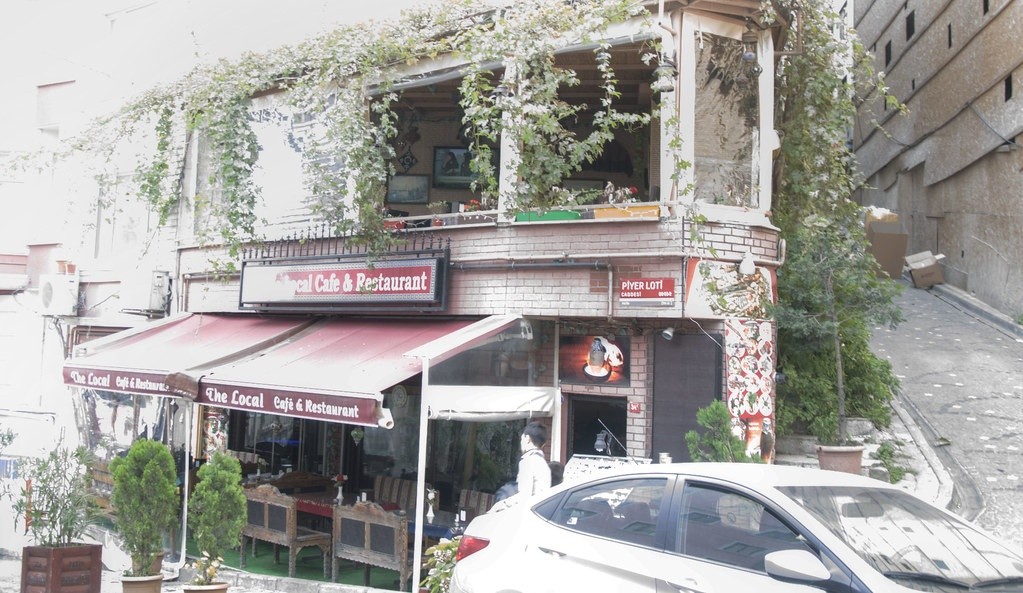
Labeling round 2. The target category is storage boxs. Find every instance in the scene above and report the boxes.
[866,222,908,278]
[902,251,946,288]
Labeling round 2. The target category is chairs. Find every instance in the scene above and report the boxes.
[760,497,804,541]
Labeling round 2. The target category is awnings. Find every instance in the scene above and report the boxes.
[61,313,517,593]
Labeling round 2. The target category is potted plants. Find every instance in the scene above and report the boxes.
[766,232,901,475]
[426,201,448,226]
[0,439,248,593]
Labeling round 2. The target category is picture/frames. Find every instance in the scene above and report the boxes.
[432,146,500,189]
[386,174,430,204]
[559,177,606,191]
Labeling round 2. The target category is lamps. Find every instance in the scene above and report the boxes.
[739,252,755,275]
[661,327,673,340]
[652,49,679,92]
[595,430,613,457]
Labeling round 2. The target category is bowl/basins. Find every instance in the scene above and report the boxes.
[583,361,612,383]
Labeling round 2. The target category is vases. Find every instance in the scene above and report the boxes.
[426,503,435,524]
[56,261,76,273]
[336,486,344,505]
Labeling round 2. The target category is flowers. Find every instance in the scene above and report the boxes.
[331,473,348,489]
[464,199,480,212]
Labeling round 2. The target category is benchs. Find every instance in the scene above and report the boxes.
[244,471,495,592]
[576,499,662,533]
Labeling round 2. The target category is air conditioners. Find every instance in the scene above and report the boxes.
[39,275,80,316]
[118,271,170,311]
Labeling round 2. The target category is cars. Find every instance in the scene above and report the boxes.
[448,461,1023,593]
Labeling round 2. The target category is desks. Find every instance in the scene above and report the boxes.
[288,491,470,561]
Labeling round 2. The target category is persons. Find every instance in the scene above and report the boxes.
[442,152,459,176]
[487,420,552,514]
[461,153,473,177]
